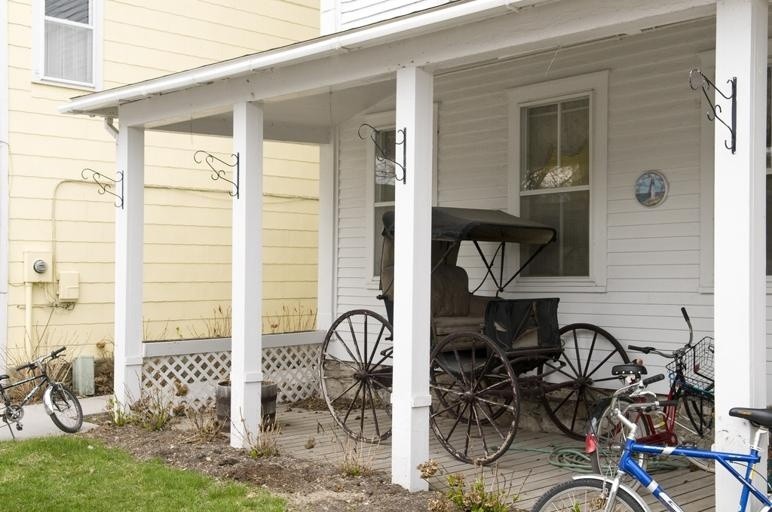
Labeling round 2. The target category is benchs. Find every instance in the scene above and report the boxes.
[383,263,504,353]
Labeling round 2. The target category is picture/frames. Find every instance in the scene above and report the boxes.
[632,169,668,209]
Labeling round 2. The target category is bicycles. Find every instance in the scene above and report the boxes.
[0,346,83,438]
[585,308,715,503]
[529,373,772,512]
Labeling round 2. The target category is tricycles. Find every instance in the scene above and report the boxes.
[319,204,635,467]
